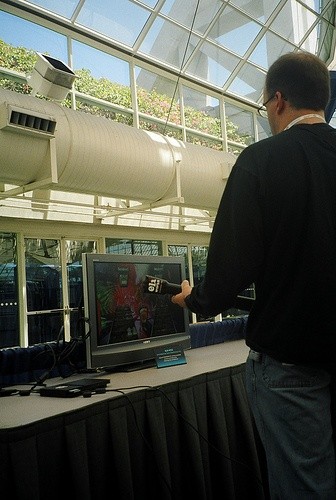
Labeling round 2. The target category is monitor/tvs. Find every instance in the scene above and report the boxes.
[83,253,191,372]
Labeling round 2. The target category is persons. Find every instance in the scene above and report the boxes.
[170,51,336,500]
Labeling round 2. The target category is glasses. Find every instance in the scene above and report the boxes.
[258,93,287,119]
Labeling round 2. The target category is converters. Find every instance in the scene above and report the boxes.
[39,385,82,398]
